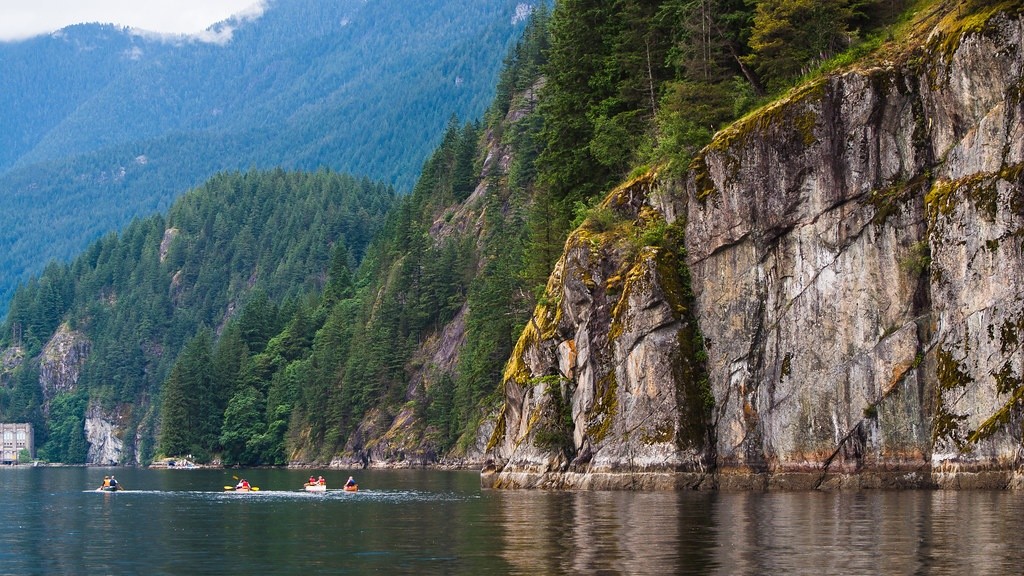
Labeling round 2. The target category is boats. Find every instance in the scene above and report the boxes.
[100,481,119,492]
[305,483,327,494]
[235,486,252,492]
[343,484,359,493]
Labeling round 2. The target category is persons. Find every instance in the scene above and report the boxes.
[109,475,120,486]
[307,475,316,486]
[100,476,111,490]
[344,476,354,487]
[310,475,325,485]
[237,478,250,488]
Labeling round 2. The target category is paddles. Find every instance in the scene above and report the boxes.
[224,485,260,492]
[232,476,241,482]
